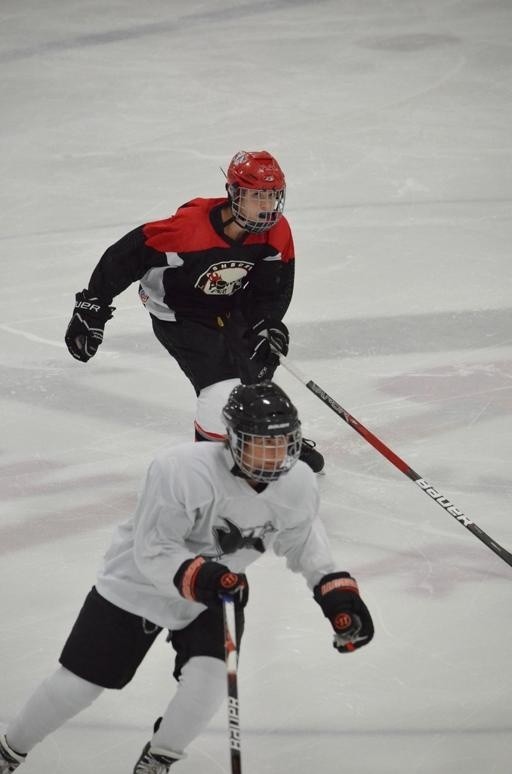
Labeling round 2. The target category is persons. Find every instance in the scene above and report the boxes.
[61,149,327,474]
[0,381,376,772]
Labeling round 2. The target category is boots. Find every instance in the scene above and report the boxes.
[0,735,27,774]
[133,714,188,774]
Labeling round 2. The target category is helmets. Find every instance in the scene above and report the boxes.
[225,149,284,192]
[221,378,301,440]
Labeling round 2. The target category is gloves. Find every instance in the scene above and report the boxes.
[236,316,291,386]
[62,286,119,365]
[312,567,374,654]
[173,556,249,610]
